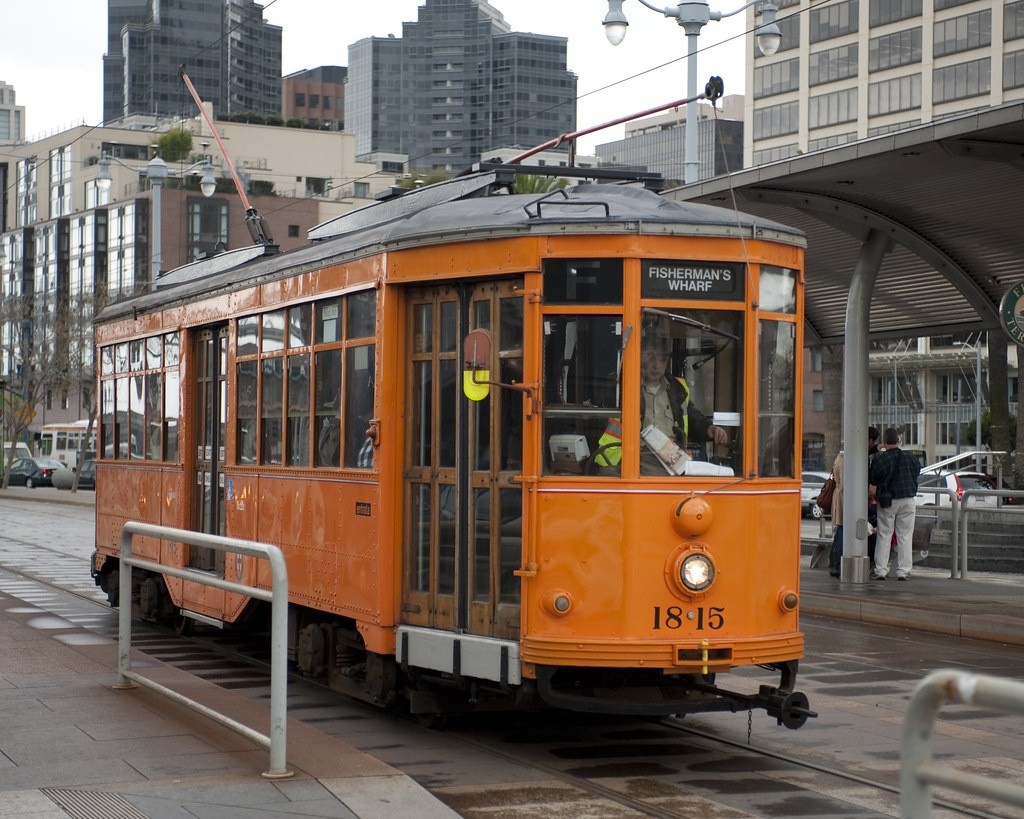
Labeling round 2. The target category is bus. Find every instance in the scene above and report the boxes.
[87,72,821,738]
[38,418,105,491]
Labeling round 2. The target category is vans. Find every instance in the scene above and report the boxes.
[0,440,36,467]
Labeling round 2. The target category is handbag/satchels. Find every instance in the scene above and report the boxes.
[878,493,891,508]
[816,468,837,515]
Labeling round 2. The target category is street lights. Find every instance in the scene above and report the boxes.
[600,0,785,184]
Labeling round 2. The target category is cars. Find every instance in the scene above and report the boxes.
[800,470,837,520]
[913,469,964,509]
[955,471,1017,509]
[0,455,69,491]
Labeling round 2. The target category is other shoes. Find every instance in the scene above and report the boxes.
[871,573,886,580]
[897,574,909,581]
[830,566,840,577]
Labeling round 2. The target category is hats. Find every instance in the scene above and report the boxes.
[882,428,898,441]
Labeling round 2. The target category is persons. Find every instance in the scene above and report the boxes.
[348,388,375,468]
[868,426,921,581]
[319,387,351,467]
[828,450,843,578]
[622,332,728,444]
